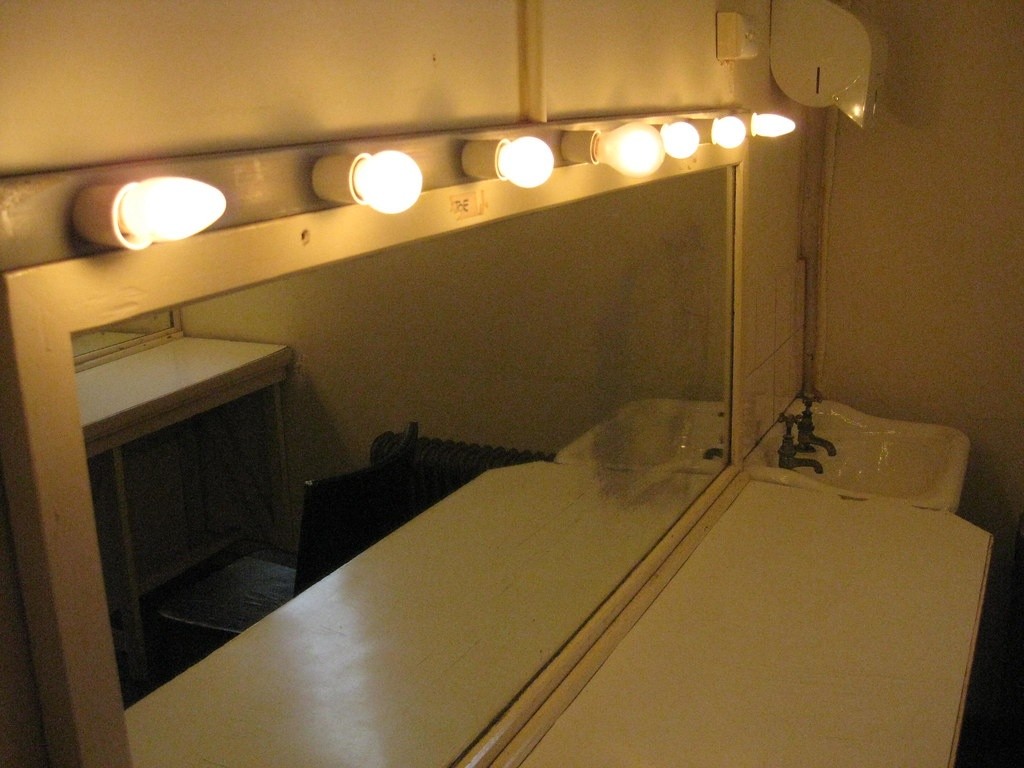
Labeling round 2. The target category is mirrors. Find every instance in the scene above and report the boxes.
[1,129,748,768]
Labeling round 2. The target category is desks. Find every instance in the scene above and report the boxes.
[73,336,292,681]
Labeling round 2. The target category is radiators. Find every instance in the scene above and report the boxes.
[372,431,556,543]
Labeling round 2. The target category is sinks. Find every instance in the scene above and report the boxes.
[742,392,971,512]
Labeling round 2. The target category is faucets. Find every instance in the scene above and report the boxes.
[776,392,837,475]
